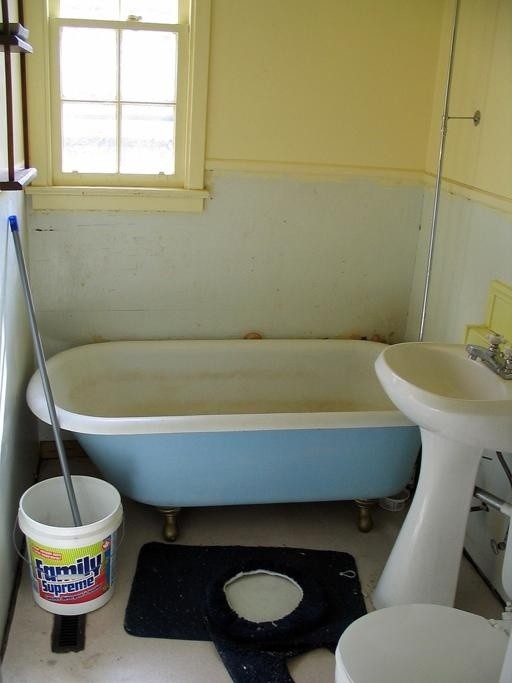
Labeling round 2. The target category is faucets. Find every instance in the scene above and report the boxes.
[465,331,511,382]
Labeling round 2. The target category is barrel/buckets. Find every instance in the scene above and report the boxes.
[13,471,127,619]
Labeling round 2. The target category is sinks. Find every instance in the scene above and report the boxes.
[378,343,511,419]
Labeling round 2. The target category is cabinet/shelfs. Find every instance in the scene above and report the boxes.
[0,0,39,192]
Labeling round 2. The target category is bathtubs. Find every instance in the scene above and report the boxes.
[21,335,423,541]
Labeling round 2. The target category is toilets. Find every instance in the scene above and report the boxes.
[333,518,511,683]
